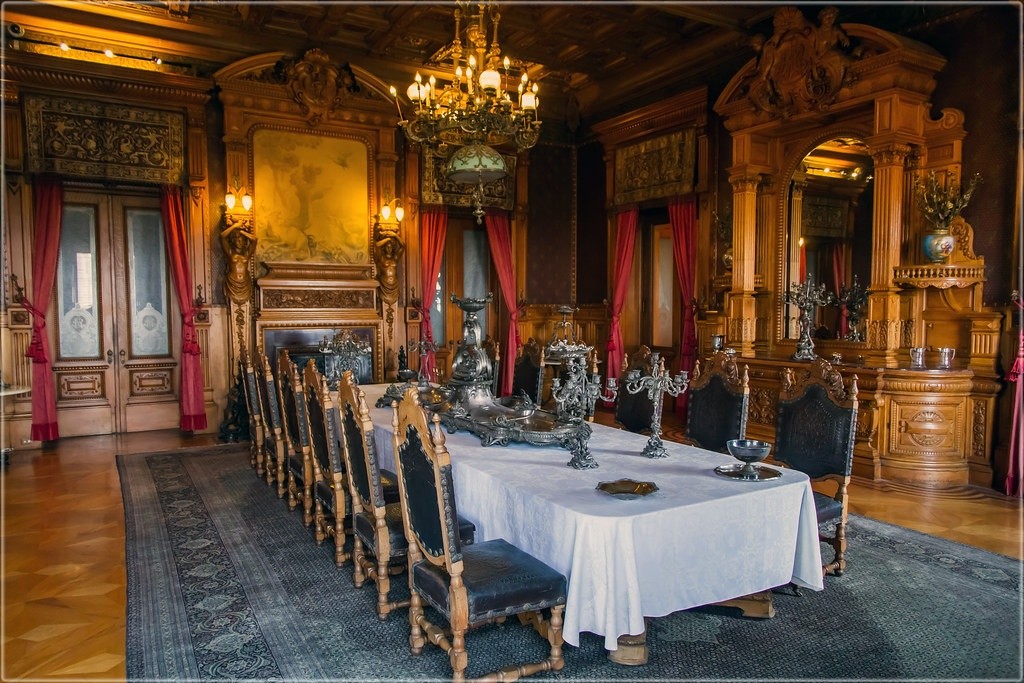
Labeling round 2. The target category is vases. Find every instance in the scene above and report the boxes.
[922,229,955,264]
[721,244,734,273]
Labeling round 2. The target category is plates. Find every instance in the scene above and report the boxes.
[595,477,660,502]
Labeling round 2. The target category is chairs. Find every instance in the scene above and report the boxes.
[241,329,858,683]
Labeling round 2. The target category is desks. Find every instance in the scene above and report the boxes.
[326,383,824,665]
[543,359,603,402]
[0,386,32,471]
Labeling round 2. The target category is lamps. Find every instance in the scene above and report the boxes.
[152,56,162,64]
[388,0,543,224]
[225,178,253,210]
[382,188,405,221]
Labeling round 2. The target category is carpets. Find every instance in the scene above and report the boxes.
[117,443,1024,683]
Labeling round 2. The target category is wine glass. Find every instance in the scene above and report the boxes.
[398,370,417,385]
[727,440,772,478]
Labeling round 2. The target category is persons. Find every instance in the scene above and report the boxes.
[219,214,258,304]
[373,233,405,306]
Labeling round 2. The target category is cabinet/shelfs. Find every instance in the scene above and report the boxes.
[709,7,1003,490]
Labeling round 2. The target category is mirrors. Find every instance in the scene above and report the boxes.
[780,137,873,343]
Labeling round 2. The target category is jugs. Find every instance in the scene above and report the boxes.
[909,347,927,370]
[937,348,956,370]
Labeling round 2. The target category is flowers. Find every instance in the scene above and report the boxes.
[915,167,982,228]
[713,204,734,246]
